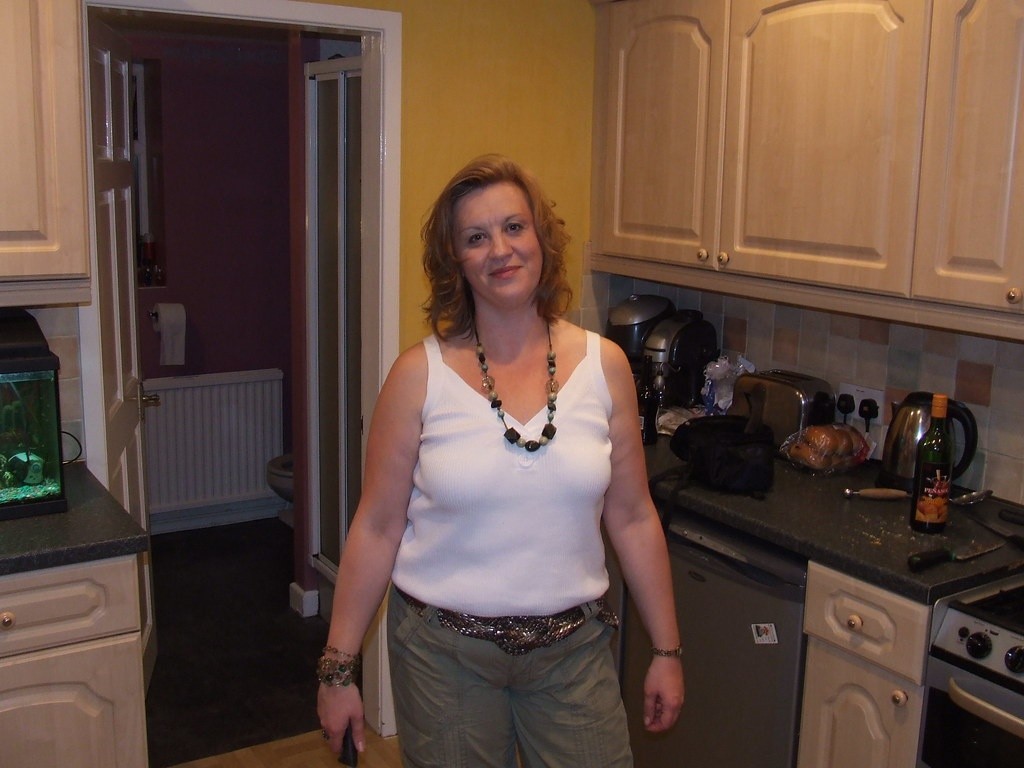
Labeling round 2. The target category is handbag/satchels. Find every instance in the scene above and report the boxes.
[688,431,773,492]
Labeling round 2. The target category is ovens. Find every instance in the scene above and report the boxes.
[916,655,1024,768]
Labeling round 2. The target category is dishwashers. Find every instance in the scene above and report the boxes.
[622,513,808,767]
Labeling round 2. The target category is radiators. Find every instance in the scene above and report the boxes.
[141,369,294,534]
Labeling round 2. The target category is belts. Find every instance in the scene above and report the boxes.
[395,585,620,656]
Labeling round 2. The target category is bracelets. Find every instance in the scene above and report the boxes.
[652,645,682,657]
[317,646,360,688]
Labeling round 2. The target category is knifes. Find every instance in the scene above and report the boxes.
[908,538,1008,570]
[962,511,1024,551]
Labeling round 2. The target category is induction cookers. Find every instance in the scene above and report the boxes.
[931,583,1024,697]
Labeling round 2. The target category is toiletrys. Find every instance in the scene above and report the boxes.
[142,264,166,287]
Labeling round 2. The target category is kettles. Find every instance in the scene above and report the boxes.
[880,390,978,491]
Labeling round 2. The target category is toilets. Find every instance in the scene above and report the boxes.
[266,452,295,530]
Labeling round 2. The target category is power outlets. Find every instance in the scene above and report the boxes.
[837,383,886,424]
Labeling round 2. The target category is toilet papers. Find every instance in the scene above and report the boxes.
[151,302,186,366]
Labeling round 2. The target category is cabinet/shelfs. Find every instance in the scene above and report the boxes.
[798,559,933,767]
[0,552,150,768]
[0,0,93,308]
[583,0,1024,343]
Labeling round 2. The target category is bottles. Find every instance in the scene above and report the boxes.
[636,356,657,447]
[908,393,954,535]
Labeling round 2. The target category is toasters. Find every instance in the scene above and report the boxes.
[734,370,836,458]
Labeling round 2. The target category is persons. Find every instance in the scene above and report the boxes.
[316,153,685,768]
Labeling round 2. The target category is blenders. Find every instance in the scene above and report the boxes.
[604,293,720,448]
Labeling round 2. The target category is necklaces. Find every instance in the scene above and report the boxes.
[475,318,556,453]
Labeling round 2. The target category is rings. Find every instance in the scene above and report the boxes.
[321,731,328,738]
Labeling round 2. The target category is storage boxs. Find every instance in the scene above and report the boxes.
[0,307,70,521]
